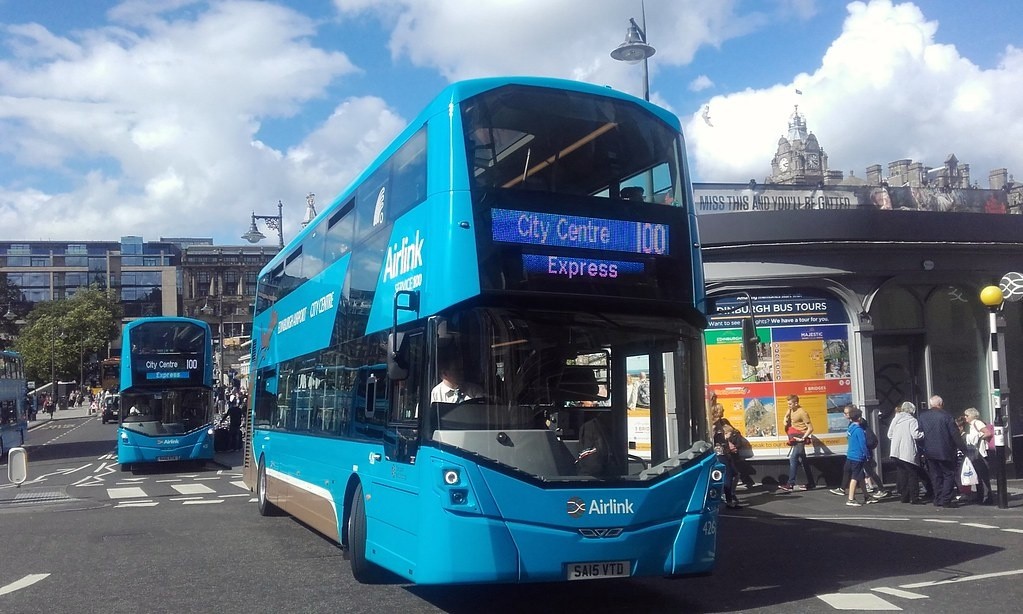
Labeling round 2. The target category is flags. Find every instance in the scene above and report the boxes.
[796,89,802,95]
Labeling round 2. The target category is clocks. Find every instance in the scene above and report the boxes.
[806,154,819,169]
[778,157,788,173]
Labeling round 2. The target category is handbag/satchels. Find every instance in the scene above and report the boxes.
[960,456,979,486]
[730,434,754,458]
[785,409,791,432]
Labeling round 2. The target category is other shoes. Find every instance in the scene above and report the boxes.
[984,499,993,505]
[971,498,983,504]
[942,502,959,508]
[914,500,926,505]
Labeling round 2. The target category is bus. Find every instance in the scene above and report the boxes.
[118,317,214,471]
[0,350,25,459]
[244,78,760,585]
[101,358,120,390]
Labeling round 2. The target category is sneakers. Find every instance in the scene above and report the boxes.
[829,488,845,496]
[799,484,816,491]
[864,496,879,504]
[778,483,793,492]
[873,490,888,498]
[845,499,863,507]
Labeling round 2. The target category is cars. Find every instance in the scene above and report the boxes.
[102,397,119,424]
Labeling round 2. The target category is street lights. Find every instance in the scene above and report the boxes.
[980,285,1009,509]
[201,297,224,387]
[241,210,285,253]
[611,17,656,104]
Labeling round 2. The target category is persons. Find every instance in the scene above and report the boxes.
[710,392,742,510]
[755,362,773,382]
[413,356,484,433]
[870,187,893,210]
[69,383,248,454]
[829,395,995,507]
[778,394,817,491]
[626,372,650,410]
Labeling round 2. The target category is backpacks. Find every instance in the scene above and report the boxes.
[861,418,878,450]
[974,419,995,450]
